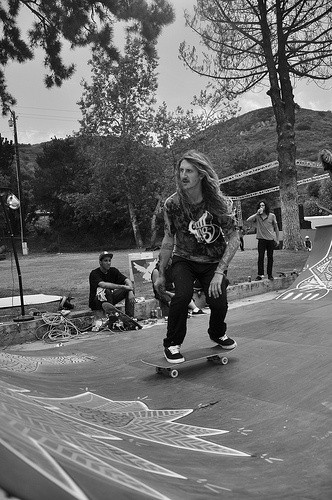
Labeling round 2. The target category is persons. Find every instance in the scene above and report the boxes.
[154,150,242,364]
[238,226,245,252]
[304,235,312,251]
[151,253,206,314]
[246,199,280,281]
[89,252,138,330]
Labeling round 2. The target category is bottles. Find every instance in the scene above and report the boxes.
[260,208,263,214]
[157,307,162,318]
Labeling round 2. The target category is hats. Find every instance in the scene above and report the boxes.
[98,251,114,260]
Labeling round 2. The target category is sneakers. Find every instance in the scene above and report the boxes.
[162,345,185,364]
[209,333,237,349]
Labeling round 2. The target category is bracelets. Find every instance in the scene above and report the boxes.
[214,271,226,276]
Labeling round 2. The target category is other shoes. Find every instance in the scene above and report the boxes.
[268,275,274,280]
[255,276,263,281]
[130,317,138,322]
[192,309,207,316]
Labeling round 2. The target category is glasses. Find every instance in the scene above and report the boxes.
[259,205,266,207]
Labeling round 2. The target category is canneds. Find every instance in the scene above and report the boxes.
[248,275,251,282]
[151,309,158,319]
[260,208,263,214]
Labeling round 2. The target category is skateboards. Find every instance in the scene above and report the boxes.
[139,344,239,378]
[102,302,143,330]
